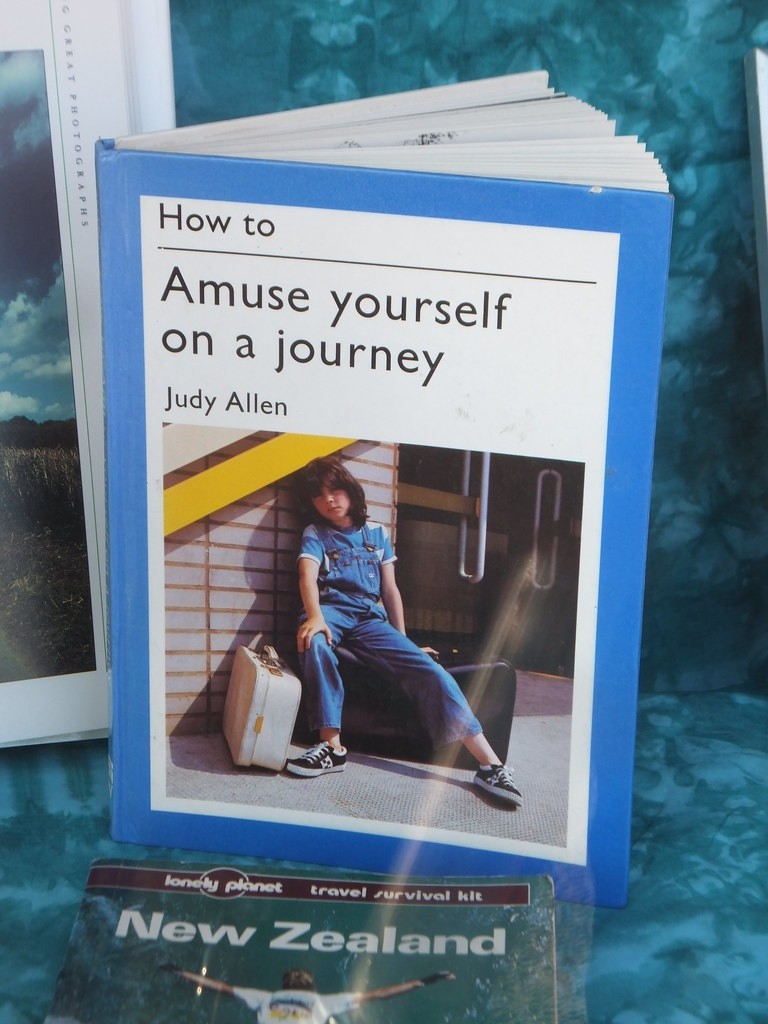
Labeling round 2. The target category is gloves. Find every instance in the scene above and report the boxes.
[419,971,456,986]
[161,963,182,976]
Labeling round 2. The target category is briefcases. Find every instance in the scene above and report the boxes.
[224,629,302,770]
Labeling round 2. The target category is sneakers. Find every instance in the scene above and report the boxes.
[475,763,522,807]
[287,734,348,777]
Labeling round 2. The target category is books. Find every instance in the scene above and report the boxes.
[42,67,675,1024]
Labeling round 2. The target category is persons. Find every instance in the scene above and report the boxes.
[161,963,456,1022]
[287,457,523,807]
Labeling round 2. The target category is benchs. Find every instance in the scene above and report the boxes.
[296,648,517,773]
